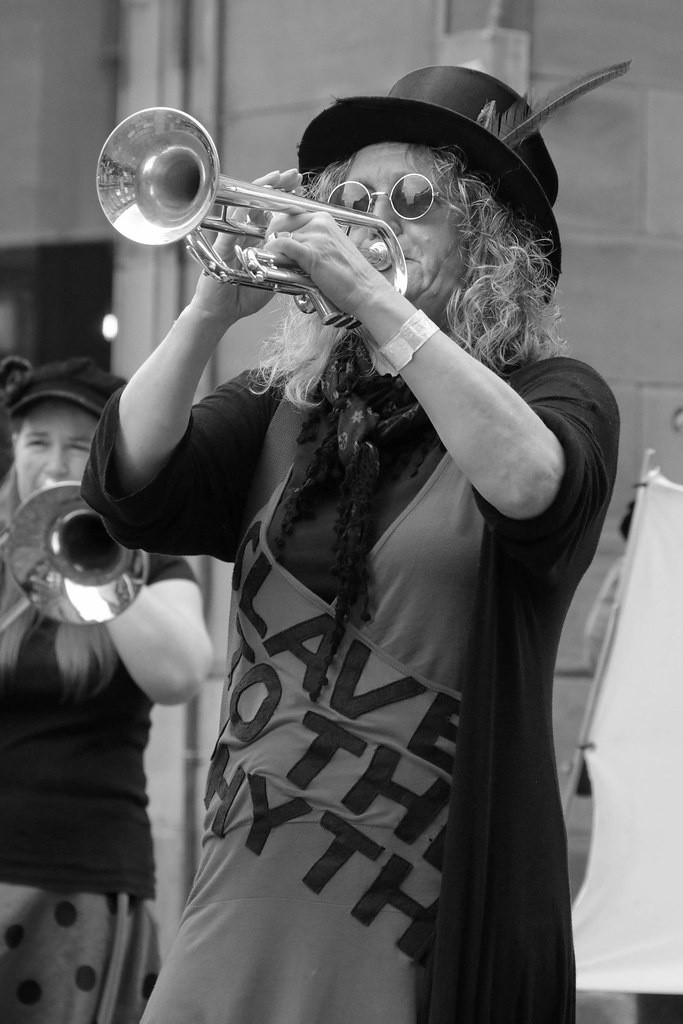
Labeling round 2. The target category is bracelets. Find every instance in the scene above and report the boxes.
[380,310,439,371]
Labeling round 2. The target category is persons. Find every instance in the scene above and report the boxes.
[82,65,620,1024]
[0,359,212,1024]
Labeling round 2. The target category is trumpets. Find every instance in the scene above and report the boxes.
[95,105,410,332]
[7,477,151,627]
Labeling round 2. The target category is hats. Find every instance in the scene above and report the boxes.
[5,356,128,418]
[300,65,562,306]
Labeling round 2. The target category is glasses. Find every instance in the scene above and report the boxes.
[327,173,469,223]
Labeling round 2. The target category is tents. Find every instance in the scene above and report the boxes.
[572,474,683,996]
